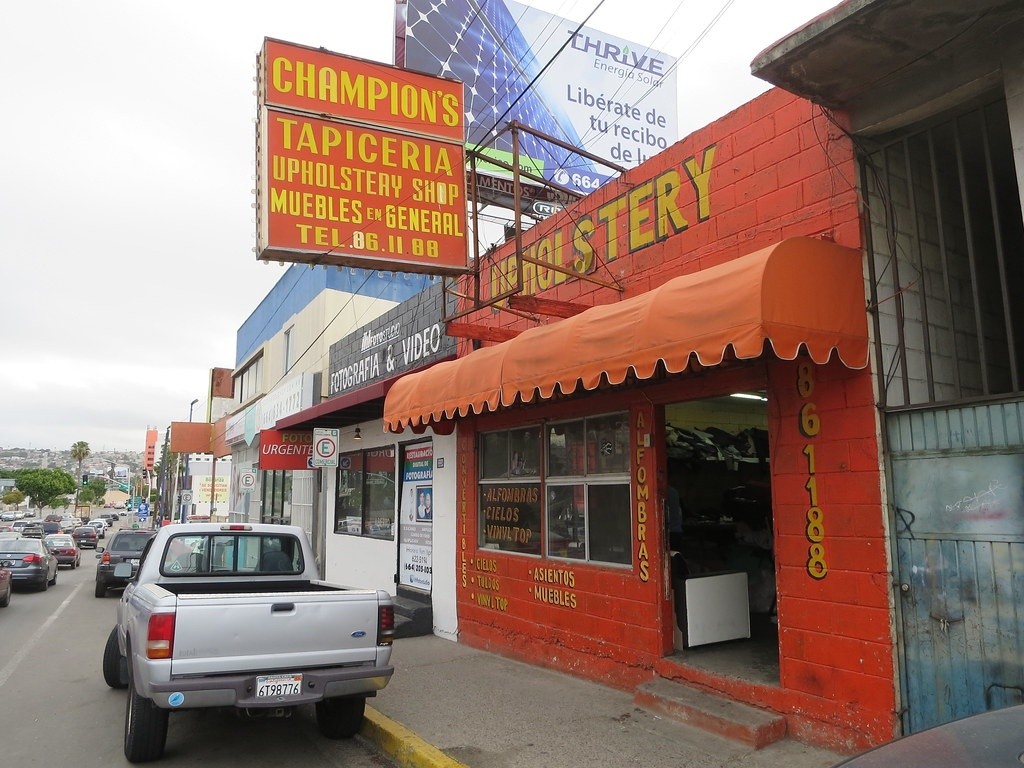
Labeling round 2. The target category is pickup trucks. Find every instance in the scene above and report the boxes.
[103,521,395,764]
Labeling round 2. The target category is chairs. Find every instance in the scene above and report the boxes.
[168,553,207,572]
[117,542,130,550]
[48,541,54,546]
[255,551,294,571]
[64,543,71,546]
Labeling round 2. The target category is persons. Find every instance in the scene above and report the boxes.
[418,490,430,519]
[156,517,160,527]
[667,459,683,550]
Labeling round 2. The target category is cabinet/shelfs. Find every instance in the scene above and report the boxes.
[666,454,772,548]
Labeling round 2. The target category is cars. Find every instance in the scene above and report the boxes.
[0,536,60,592]
[41,534,83,569]
[0,502,132,548]
[69,525,102,550]
[335,491,366,524]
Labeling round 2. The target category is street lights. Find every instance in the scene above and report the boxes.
[181,398,199,523]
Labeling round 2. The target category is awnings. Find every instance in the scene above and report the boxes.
[383,235,869,435]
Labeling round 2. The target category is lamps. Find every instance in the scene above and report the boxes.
[354,427,361,440]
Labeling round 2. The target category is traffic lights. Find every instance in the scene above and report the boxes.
[83,474,88,485]
[143,466,148,478]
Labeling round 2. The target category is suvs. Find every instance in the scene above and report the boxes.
[95,527,159,599]
